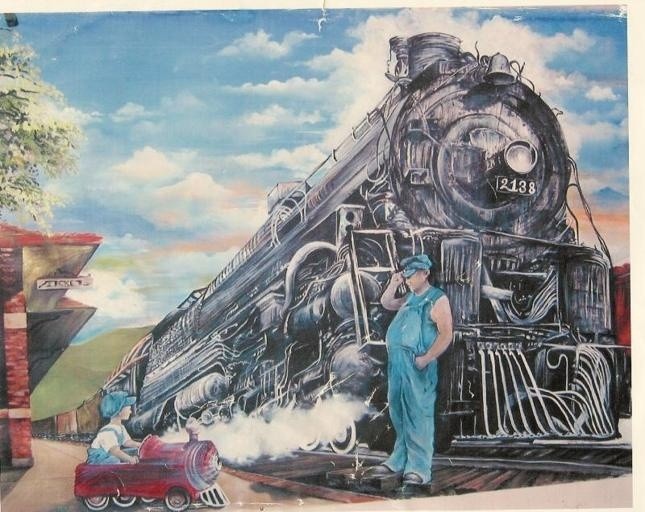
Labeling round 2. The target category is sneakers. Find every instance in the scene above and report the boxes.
[403,471,423,486]
[369,465,394,476]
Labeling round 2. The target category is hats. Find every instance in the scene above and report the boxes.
[400,253,433,278]
[99,391,137,416]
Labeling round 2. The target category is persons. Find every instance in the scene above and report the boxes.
[85,390,141,466]
[371,255,455,488]
